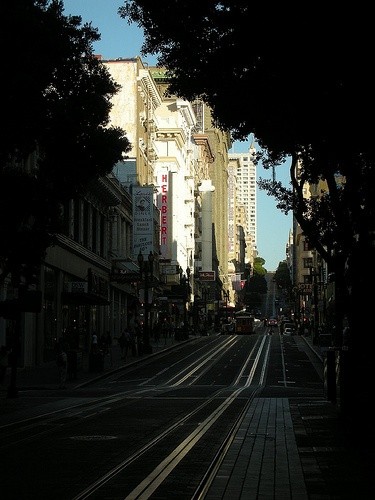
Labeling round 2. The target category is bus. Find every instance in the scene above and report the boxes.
[234,311,255,335]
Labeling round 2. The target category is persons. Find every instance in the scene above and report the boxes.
[102,331,113,343]
[118,322,161,359]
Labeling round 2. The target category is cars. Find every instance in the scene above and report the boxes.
[174,321,189,341]
[280,319,298,337]
[256,308,279,328]
[220,319,235,336]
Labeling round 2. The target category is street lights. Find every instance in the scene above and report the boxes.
[201,285,210,322]
[178,267,191,322]
[308,263,321,346]
[137,249,156,354]
